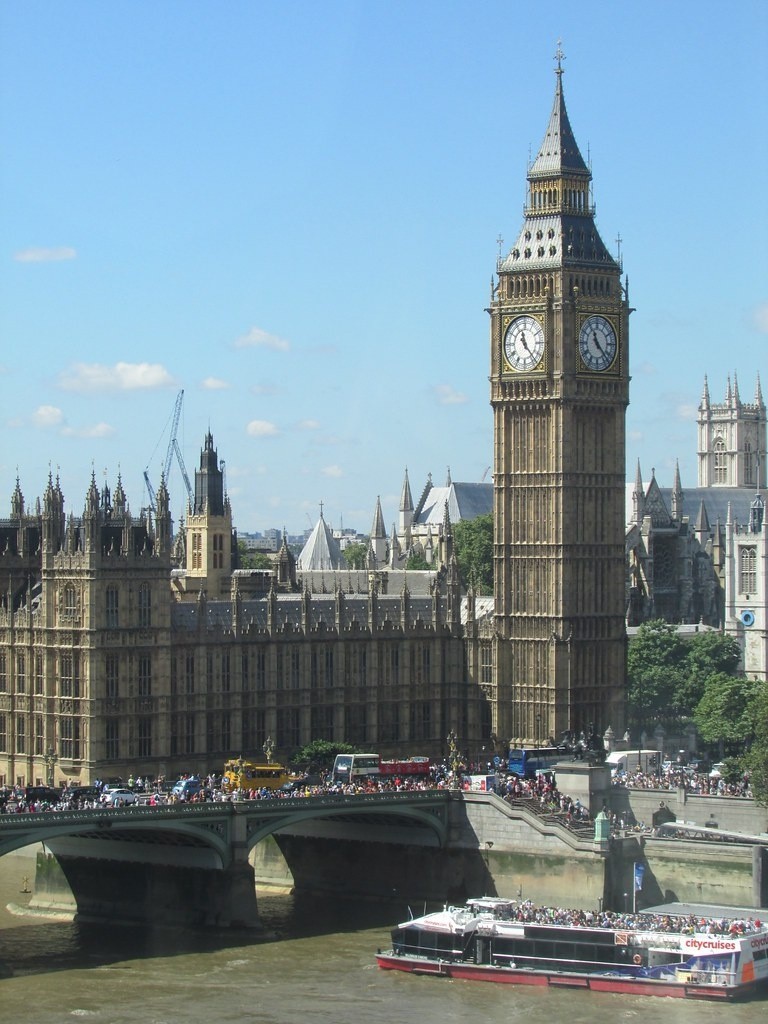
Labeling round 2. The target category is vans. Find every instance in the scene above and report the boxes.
[710,764,728,780]
[15,786,60,803]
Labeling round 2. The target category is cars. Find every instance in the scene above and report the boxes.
[283,774,324,792]
[174,780,214,800]
[63,787,97,802]
[100,789,141,805]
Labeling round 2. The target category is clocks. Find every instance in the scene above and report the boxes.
[578,314,620,375]
[501,313,547,373]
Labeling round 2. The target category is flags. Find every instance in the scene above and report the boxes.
[634,862,645,892]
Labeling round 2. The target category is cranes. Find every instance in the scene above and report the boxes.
[143,387,194,518]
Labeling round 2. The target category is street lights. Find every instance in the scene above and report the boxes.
[678,749,686,790]
[536,713,541,749]
[263,736,276,765]
[447,727,458,789]
[42,743,58,786]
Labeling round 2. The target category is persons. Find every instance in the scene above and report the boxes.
[469,758,749,838]
[0,762,467,814]
[498,901,761,939]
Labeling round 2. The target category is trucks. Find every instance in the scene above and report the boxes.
[332,752,430,787]
[606,749,663,777]
[224,760,288,793]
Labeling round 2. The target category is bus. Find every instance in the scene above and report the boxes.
[509,745,582,780]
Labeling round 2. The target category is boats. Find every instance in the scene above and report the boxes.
[377,896,766,1004]
